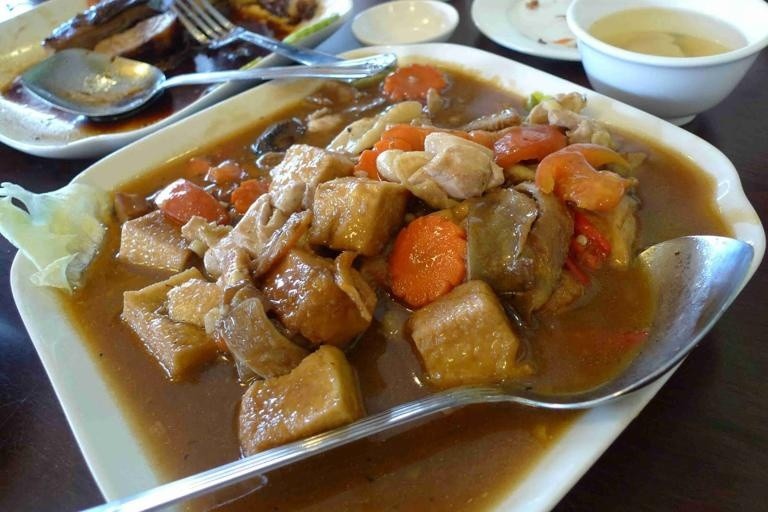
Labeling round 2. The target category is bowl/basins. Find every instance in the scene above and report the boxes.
[350,1,461,48]
[564,0,768,131]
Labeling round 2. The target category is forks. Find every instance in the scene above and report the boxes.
[171,0,357,84]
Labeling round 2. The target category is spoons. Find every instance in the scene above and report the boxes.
[92,230,757,510]
[18,47,398,119]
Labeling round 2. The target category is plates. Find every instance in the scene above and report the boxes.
[7,39,766,511]
[471,1,584,66]
[1,2,354,163]
